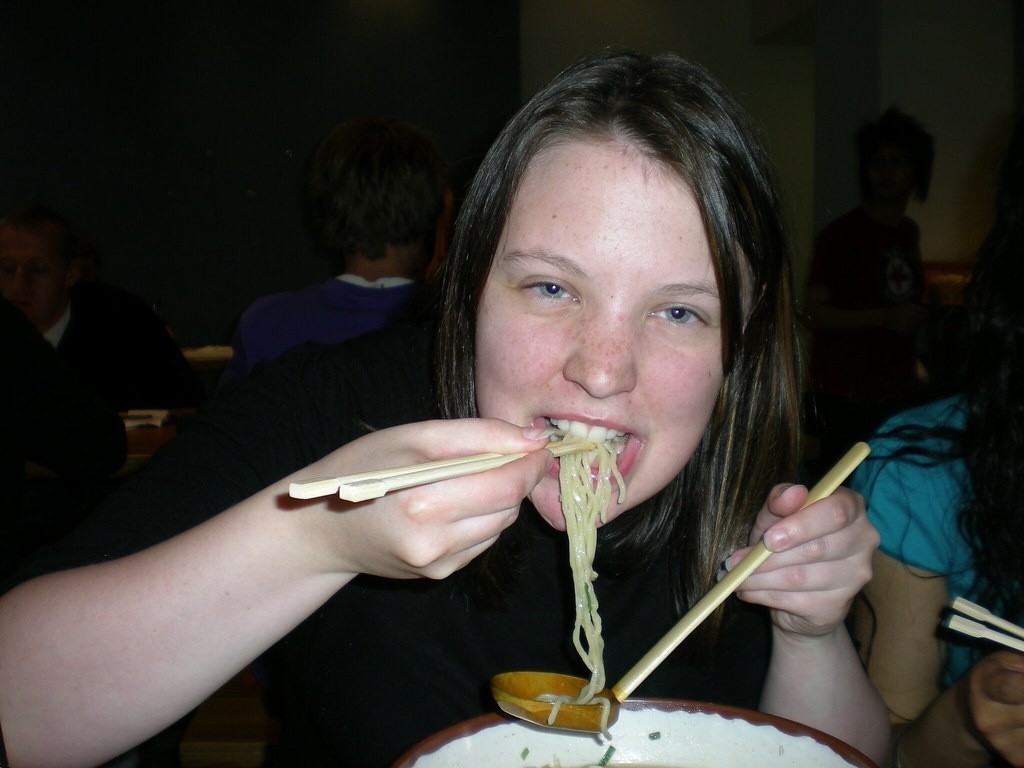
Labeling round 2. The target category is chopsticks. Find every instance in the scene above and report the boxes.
[948,597,1024,652]
[288,439,595,502]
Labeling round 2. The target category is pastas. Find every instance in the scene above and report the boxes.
[539,434,627,740]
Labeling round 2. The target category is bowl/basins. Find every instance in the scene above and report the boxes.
[391,698,881,767]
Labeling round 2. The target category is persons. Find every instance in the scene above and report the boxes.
[222,114,451,388]
[0,44,894,768]
[804,109,982,465]
[1,206,202,567]
[851,123,1024,768]
